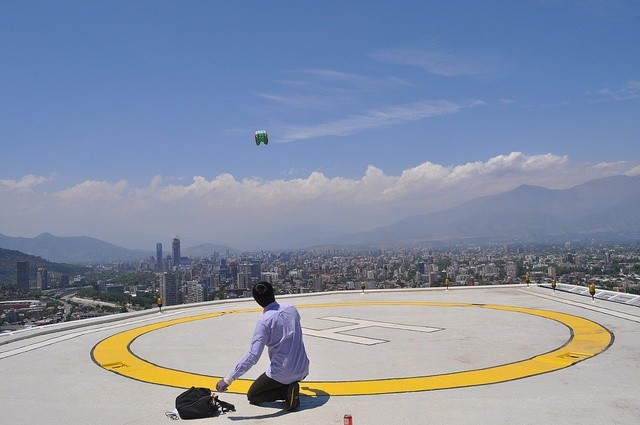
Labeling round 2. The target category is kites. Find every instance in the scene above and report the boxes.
[255,130,268,146]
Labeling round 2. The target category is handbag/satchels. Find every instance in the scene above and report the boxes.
[175,386,236,420]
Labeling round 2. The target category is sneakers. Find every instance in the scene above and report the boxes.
[287,382,300,411]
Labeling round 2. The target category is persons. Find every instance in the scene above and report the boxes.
[216,281,309,410]
